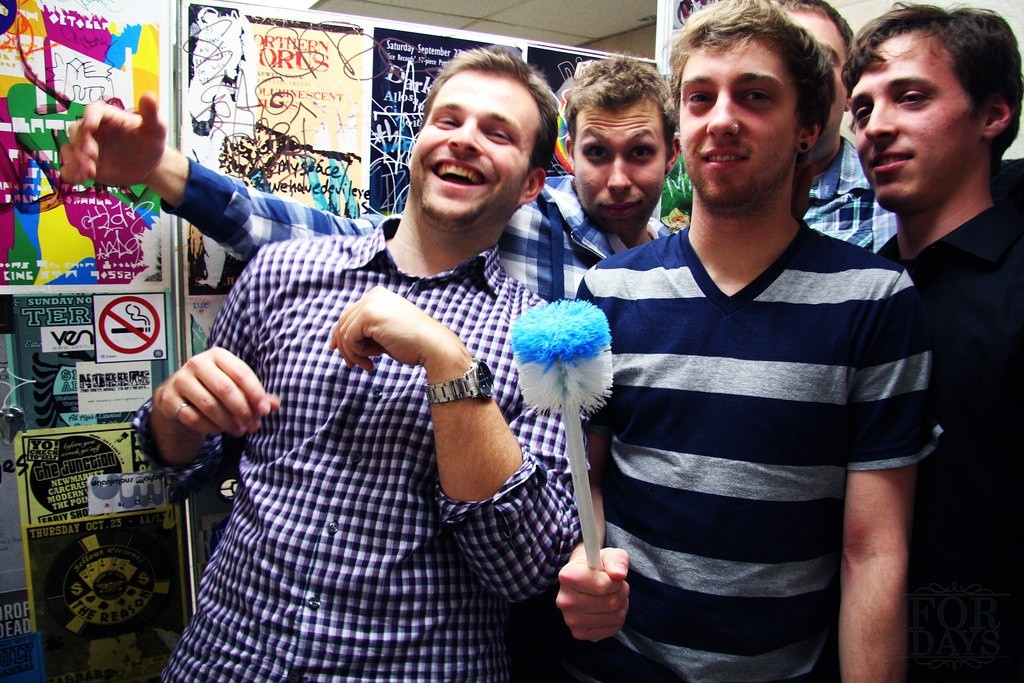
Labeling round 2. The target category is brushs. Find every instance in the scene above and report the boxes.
[509,299,615,571]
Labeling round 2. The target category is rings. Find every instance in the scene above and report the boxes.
[175,403,192,420]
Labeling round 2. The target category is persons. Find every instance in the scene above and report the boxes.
[129,44,582,683]
[59,58,680,304]
[839,5,1024,683]
[556,0,945,683]
[779,0,896,254]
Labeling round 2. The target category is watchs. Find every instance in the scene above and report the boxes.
[424,358,495,404]
